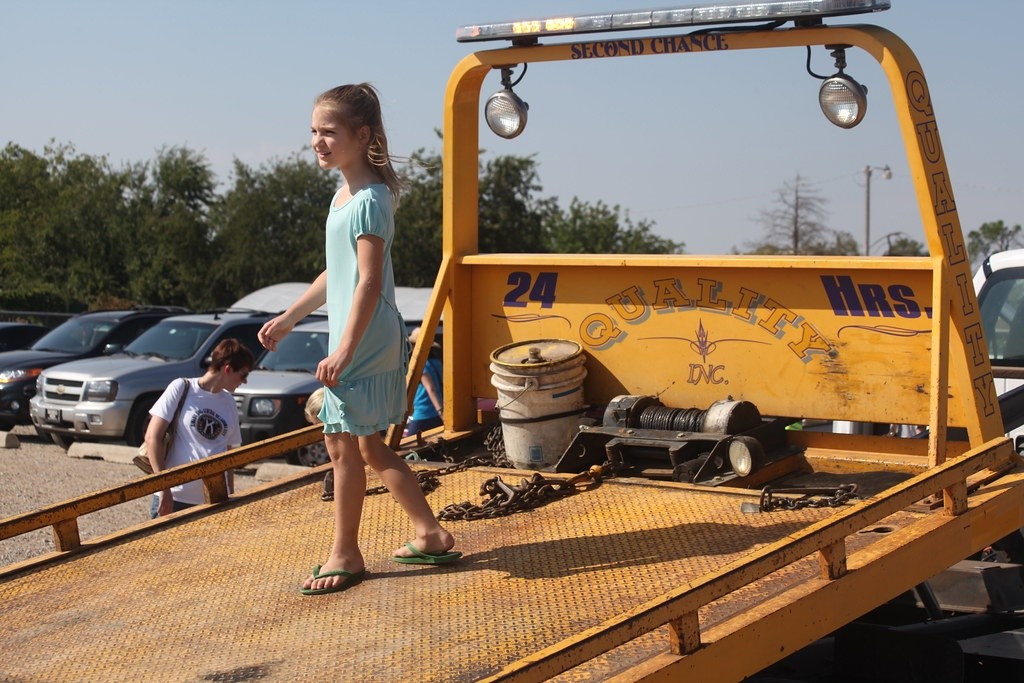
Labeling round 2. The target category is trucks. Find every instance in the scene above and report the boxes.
[1,2,1024,683]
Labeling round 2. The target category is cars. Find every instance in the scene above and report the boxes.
[233,286,443,467]
[0,322,82,353]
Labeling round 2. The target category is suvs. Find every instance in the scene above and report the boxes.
[30,282,313,448]
[0,309,193,431]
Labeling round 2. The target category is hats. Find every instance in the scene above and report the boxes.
[406,327,440,348]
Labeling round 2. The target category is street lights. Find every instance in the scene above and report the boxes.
[865,164,892,256]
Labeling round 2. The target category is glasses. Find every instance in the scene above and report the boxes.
[239,369,248,380]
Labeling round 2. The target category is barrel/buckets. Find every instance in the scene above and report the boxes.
[490,337,590,468]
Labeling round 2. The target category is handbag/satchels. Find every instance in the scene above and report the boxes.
[132,376,190,475]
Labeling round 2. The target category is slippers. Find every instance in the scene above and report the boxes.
[392,542,463,564]
[299,564,366,594]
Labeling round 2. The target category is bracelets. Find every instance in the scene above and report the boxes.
[438,408,444,415]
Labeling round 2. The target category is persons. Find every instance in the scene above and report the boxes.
[306,388,326,425]
[406,326,444,437]
[256,82,462,596]
[145,337,255,521]
